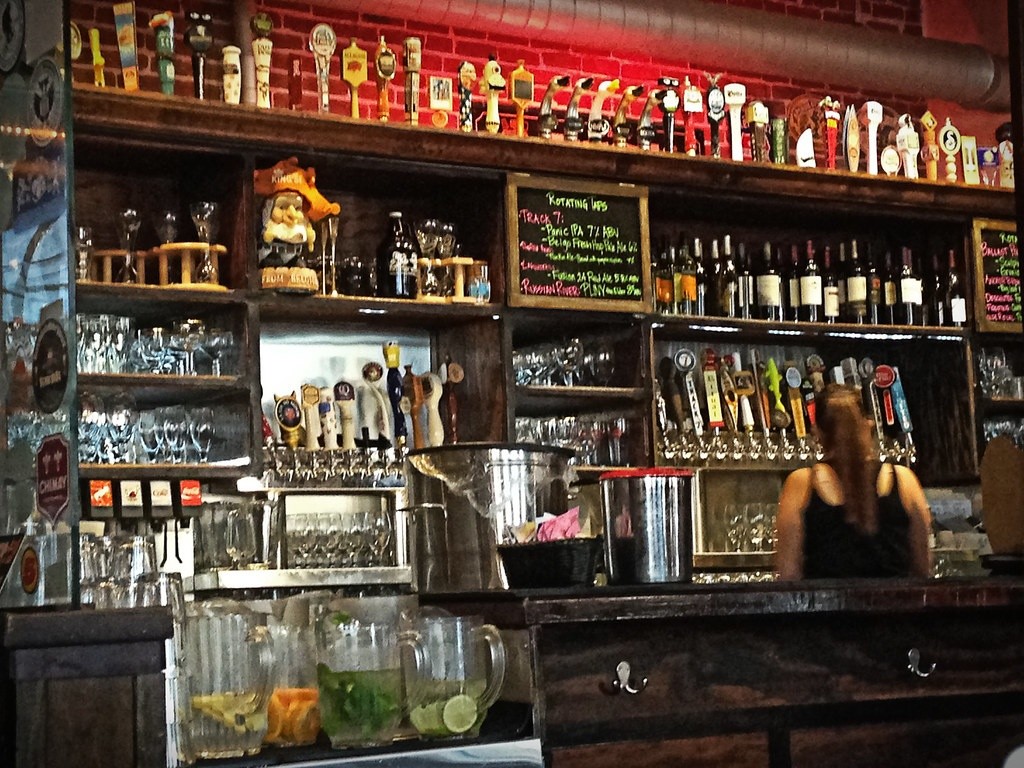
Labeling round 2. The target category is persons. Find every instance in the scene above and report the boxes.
[777,383,931,579]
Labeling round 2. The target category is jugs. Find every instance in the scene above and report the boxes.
[177,599,275,755]
[404,617,506,741]
[318,630,428,749]
[262,623,318,747]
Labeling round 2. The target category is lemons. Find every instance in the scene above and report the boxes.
[410,694,478,736]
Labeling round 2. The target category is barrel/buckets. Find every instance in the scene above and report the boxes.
[601,474,691,583]
[401,441,576,595]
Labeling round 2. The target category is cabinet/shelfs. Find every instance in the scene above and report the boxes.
[27,88,1024,598]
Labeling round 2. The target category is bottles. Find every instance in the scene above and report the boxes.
[376,212,419,298]
[649,231,966,326]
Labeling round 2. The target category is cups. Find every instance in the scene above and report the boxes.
[80,531,185,610]
[75,313,234,377]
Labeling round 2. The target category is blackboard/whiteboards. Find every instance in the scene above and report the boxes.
[972,218,1023,333]
[508,173,654,312]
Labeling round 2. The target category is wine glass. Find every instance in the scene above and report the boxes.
[113,209,141,283]
[192,202,222,284]
[151,210,179,282]
[79,395,215,465]
[286,513,392,568]
[225,514,256,570]
[723,503,778,552]
[979,346,1024,449]
[74,227,92,280]
[511,341,625,467]
[413,218,456,296]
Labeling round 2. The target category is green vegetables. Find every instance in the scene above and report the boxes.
[316,661,403,741]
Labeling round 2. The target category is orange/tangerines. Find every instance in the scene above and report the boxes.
[264,685,321,744]
